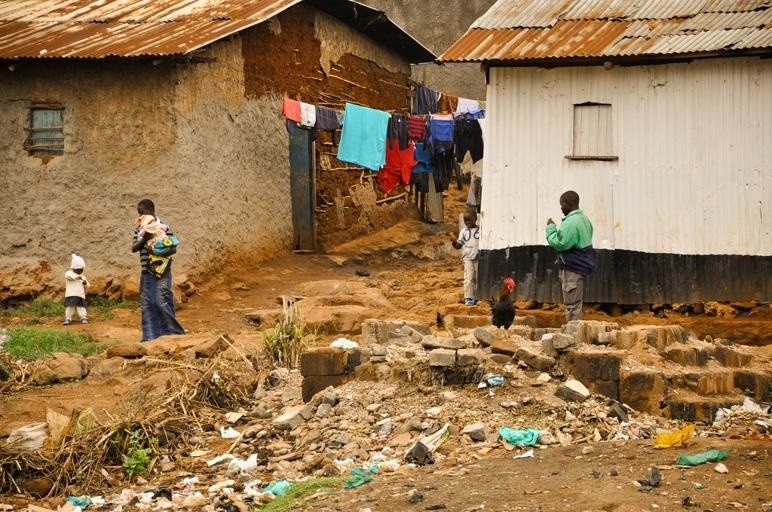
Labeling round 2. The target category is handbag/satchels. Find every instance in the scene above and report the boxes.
[152,237,179,256]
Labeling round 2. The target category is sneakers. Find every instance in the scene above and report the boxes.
[465,298,475,306]
[64,319,72,325]
[80,318,88,324]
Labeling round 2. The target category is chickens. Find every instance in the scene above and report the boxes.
[488,277,516,340]
[436,311,446,331]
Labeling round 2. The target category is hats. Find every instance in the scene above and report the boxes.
[70,254,85,270]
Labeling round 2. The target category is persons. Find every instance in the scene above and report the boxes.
[545,191,593,322]
[450,208,480,306]
[131,198,185,343]
[134,214,173,280]
[63,253,91,324]
